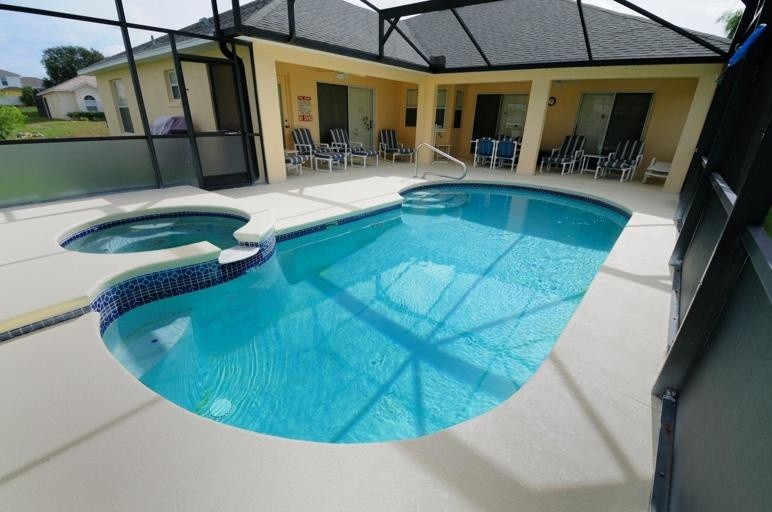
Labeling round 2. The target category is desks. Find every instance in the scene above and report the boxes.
[644,156,672,184]
[580,154,615,179]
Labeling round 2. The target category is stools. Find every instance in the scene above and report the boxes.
[436,143,453,161]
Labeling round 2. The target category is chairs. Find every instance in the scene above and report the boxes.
[539,135,587,176]
[330,127,379,167]
[284,149,307,176]
[379,128,416,164]
[594,138,645,181]
[473,134,523,171]
[291,128,348,173]
